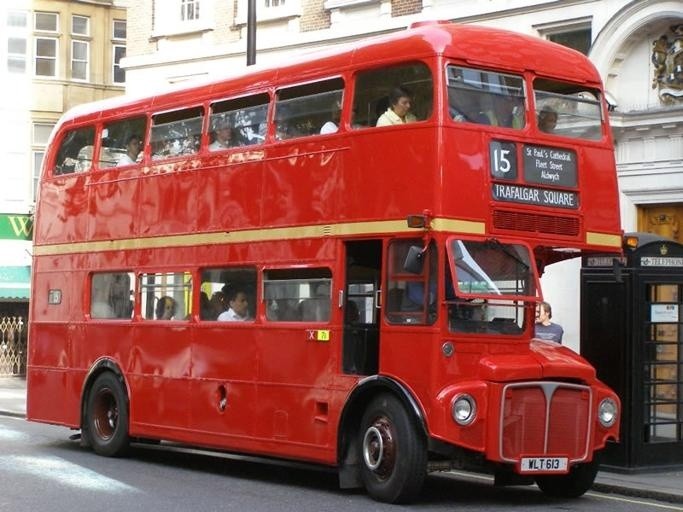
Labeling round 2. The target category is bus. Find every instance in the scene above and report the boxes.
[26,20,623,505]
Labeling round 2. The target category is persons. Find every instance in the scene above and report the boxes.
[113,133,140,168]
[400,247,451,325]
[375,86,418,127]
[216,285,255,322]
[208,116,232,151]
[536,104,557,134]
[318,90,369,135]
[473,94,523,130]
[154,296,176,320]
[534,301,563,343]
